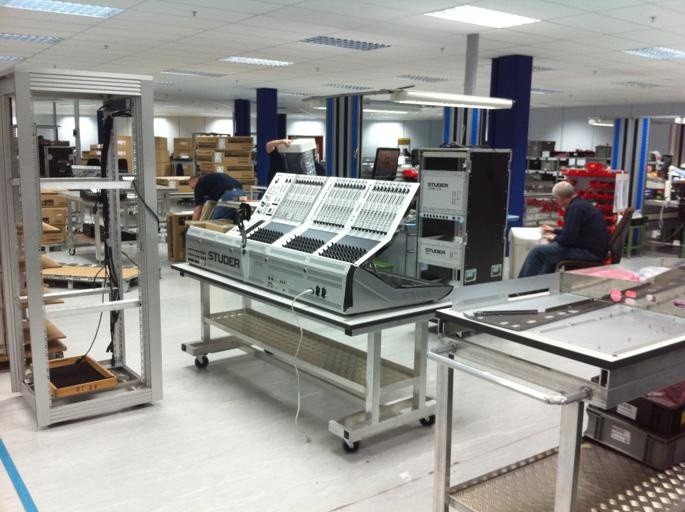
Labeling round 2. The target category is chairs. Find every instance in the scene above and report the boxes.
[554,207,634,274]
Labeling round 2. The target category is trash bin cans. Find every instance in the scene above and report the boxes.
[509,227,542,279]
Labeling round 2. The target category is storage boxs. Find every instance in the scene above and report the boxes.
[39,190,66,243]
[88,133,256,264]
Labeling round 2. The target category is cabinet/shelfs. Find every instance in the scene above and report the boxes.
[3,65,165,430]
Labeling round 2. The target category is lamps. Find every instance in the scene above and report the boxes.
[588,116,615,127]
[397,91,514,112]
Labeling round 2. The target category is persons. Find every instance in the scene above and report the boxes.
[264,138,296,188]
[514,180,609,280]
[188,172,248,220]
